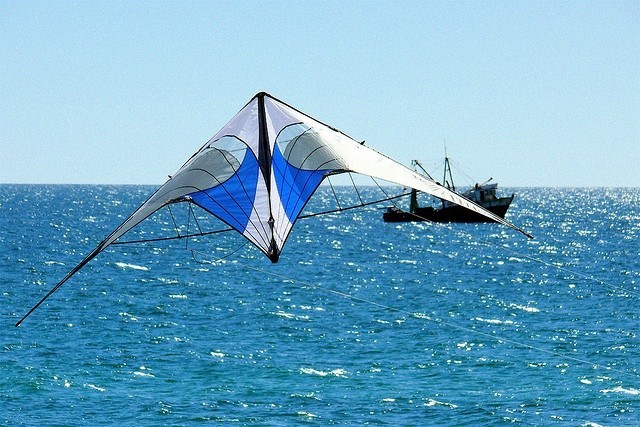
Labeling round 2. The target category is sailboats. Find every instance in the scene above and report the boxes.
[383,156,514,223]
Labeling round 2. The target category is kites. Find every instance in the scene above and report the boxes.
[14,91,535,325]
